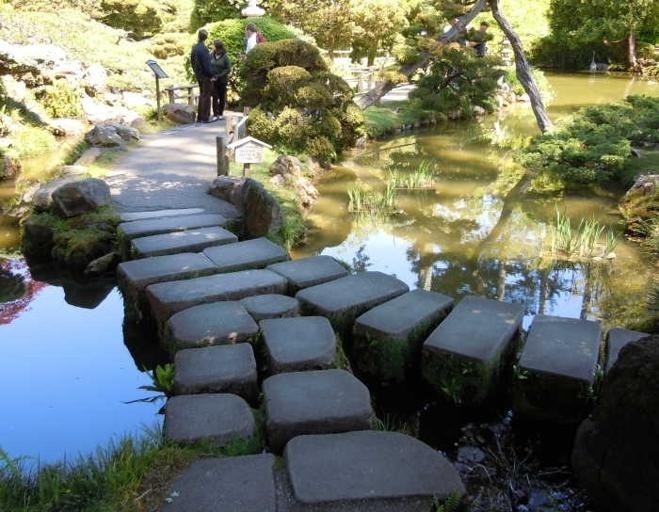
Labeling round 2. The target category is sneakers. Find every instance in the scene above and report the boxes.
[198,115,223,123]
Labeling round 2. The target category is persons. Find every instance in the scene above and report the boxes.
[245,23,266,59]
[470,21,489,57]
[191,29,214,124]
[210,40,230,121]
[453,18,467,47]
